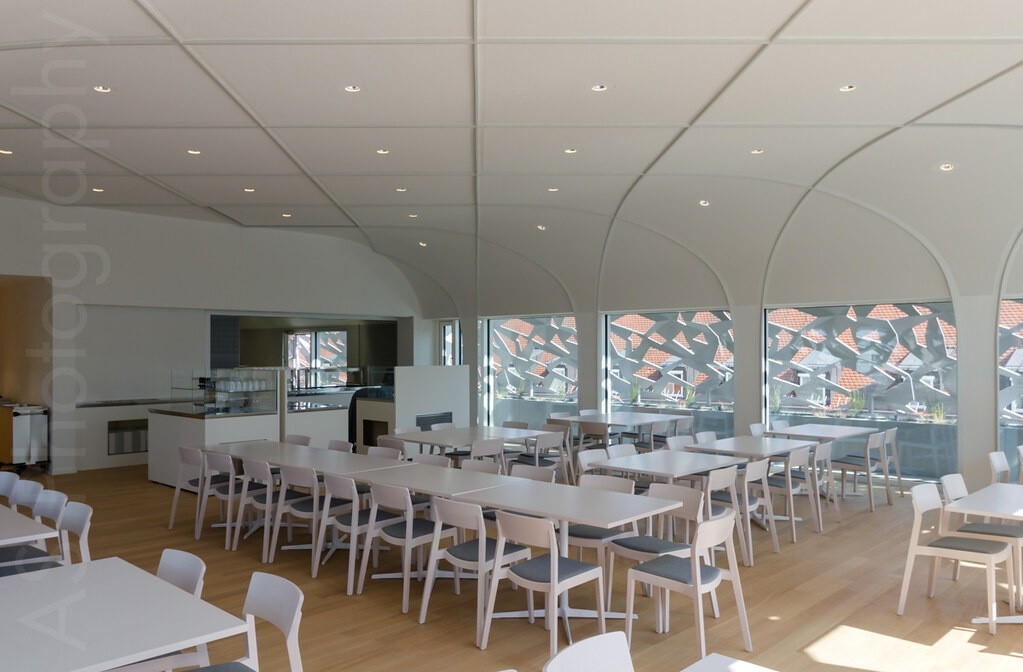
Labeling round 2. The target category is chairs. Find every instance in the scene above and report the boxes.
[113,546,210,672]
[185,572,304,672]
[984,451,1011,526]
[166,409,905,662]
[543,630,635,672]
[895,483,1016,636]
[941,474,1023,613]
[1017,446,1023,483]
[0,470,92,577]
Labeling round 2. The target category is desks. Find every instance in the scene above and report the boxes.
[686,430,822,531]
[203,439,420,560]
[356,397,395,453]
[561,411,693,442]
[451,479,684,648]
[0,503,63,567]
[393,429,504,468]
[147,405,277,494]
[0,557,249,671]
[765,422,879,503]
[0,401,48,464]
[943,481,1023,532]
[587,449,749,543]
[463,424,555,453]
[346,463,533,573]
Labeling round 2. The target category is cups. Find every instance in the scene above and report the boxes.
[227,379,266,393]
